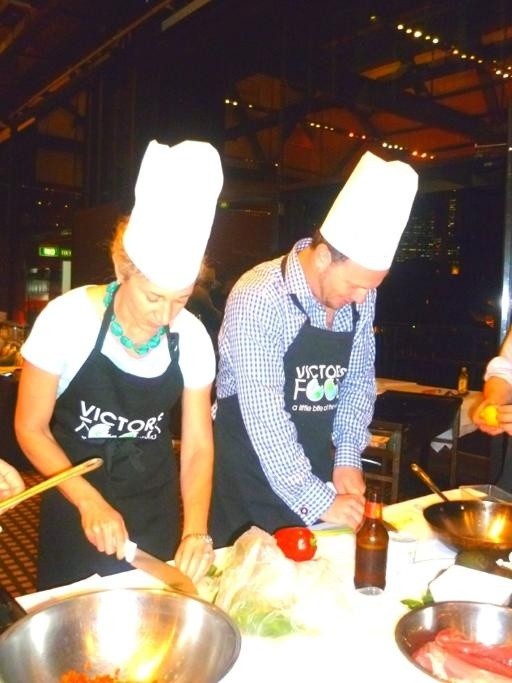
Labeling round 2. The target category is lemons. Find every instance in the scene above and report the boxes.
[481,405,500,429]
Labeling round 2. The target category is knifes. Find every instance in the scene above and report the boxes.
[118,537,199,594]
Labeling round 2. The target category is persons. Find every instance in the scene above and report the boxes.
[472,325,512,495]
[208,229,390,550]
[14,219,215,592]
[0,458,27,511]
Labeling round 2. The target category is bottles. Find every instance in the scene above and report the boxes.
[458,366,471,393]
[352,484,389,597]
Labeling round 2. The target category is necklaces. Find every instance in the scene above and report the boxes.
[103,281,166,356]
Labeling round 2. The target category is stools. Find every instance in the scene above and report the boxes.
[362,419,414,504]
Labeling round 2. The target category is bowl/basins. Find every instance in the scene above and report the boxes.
[392,599,512,680]
[1,586,243,682]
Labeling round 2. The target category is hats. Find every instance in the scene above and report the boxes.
[321,149,419,271]
[124,139,224,292]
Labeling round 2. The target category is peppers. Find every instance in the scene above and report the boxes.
[275,527,319,563]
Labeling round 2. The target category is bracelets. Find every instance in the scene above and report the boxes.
[181,533,215,546]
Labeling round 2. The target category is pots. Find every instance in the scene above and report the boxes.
[409,457,511,562]
